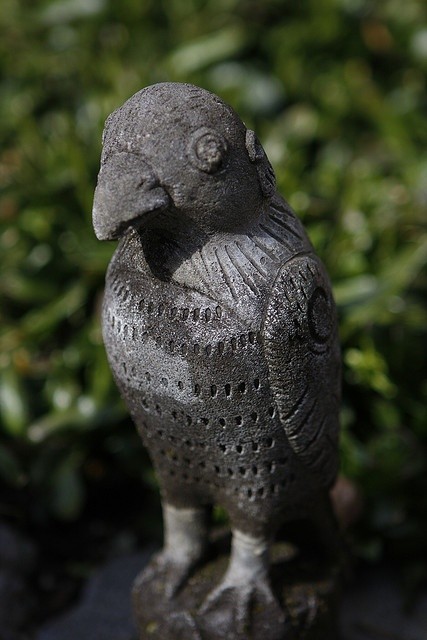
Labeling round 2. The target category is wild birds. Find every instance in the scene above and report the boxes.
[90,79,345,633]
